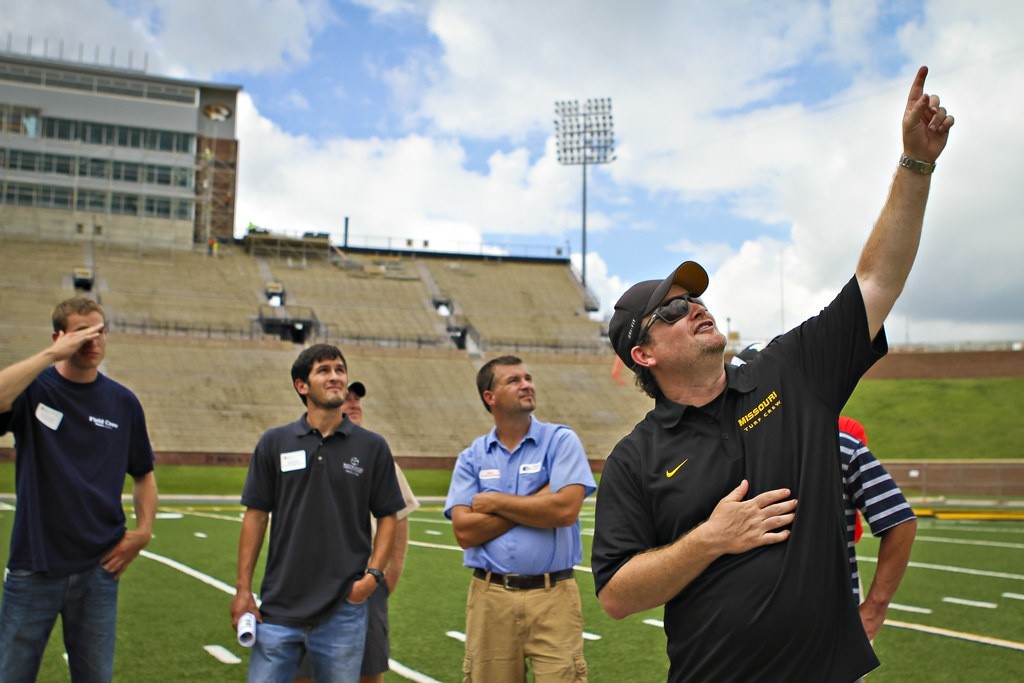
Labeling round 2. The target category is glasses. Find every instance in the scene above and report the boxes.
[636,292,706,345]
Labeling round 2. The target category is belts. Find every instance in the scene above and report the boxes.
[473,567,573,591]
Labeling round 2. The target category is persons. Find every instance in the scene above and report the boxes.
[230,343,406,683]
[590,65,955,683]
[0,297,158,683]
[443,355,596,683]
[838,414,917,644]
[208,237,218,256]
[342,381,420,682]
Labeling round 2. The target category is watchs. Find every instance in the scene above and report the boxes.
[367,568,384,585]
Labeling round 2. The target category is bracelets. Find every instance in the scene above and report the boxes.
[899,153,936,175]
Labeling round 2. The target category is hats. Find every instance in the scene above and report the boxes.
[608,260,709,373]
[347,381,366,399]
[730,342,768,367]
